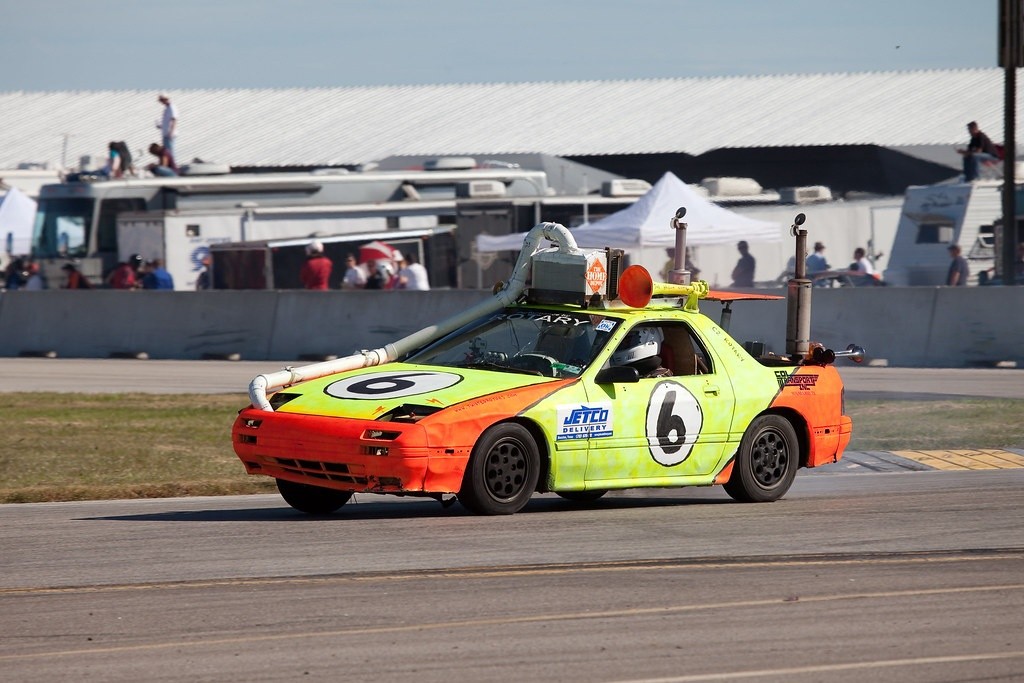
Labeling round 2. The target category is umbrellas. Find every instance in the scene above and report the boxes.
[359,240,405,290]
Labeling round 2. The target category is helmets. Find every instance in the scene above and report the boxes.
[130,254,143,266]
[158,95,169,102]
[305,241,324,255]
[610,326,664,366]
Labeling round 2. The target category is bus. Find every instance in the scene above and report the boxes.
[0,152,1024,289]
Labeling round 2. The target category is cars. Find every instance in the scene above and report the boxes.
[230,299,855,525]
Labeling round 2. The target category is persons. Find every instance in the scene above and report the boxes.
[956,121,1002,183]
[299,239,431,291]
[977,271,993,286]
[0,241,173,292]
[145,94,183,178]
[786,242,875,287]
[944,244,968,287]
[658,246,701,285]
[727,240,756,289]
[609,323,674,379]
[57,141,140,182]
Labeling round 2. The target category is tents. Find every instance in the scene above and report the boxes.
[475,171,783,289]
